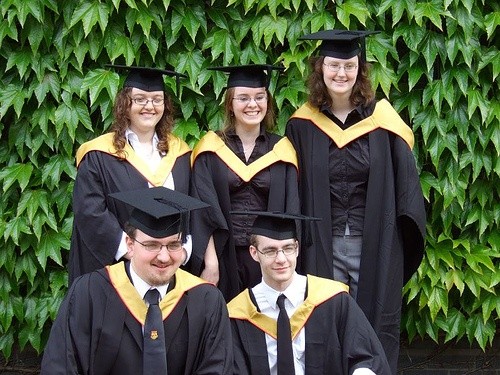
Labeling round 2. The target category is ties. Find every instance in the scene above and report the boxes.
[276,294,295,375]
[142,288,168,375]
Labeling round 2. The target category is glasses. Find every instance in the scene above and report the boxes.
[231,95,269,102]
[323,62,359,71]
[130,97,165,105]
[253,242,298,257]
[128,233,183,252]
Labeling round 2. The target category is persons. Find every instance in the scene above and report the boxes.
[39,186,232,375]
[226,212,391,375]
[190,65,301,302]
[287,29,427,375]
[68,65,192,287]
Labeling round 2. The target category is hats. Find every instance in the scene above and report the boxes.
[296,29,381,69]
[206,64,286,88]
[229,210,322,248]
[103,64,188,99]
[107,186,213,244]
